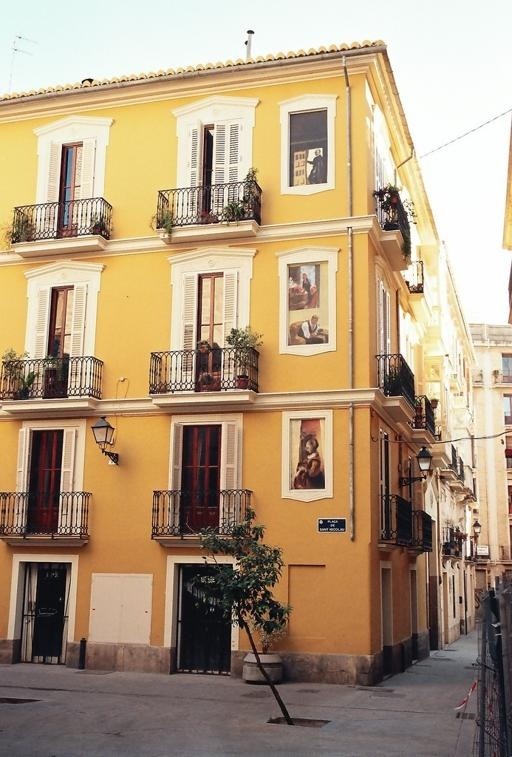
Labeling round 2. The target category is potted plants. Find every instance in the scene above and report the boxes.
[90,211,115,238]
[221,168,262,221]
[198,324,266,390]
[189,503,293,684]
[0,345,60,403]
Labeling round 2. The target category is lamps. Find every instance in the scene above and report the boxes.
[469,520,482,540]
[90,416,118,469]
[399,444,434,489]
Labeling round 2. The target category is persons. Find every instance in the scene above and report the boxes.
[296,437,324,489]
[296,313,323,345]
[300,273,314,309]
[305,149,326,185]
